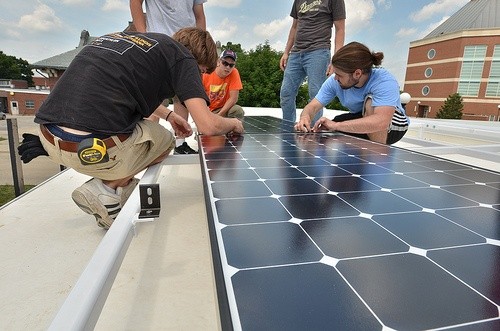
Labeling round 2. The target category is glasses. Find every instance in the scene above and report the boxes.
[220,59,235,68]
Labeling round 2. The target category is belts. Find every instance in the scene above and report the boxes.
[40,124,131,152]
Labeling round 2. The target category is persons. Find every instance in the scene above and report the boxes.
[294,42,410,145]
[33,27,243,230]
[198,50,245,133]
[130,0,208,155]
[280,0,346,127]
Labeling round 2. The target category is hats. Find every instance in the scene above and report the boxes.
[220,50,237,60]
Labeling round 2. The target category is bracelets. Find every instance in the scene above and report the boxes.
[165,110,174,121]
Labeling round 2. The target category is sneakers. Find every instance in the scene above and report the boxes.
[72,178,140,230]
[174,142,198,154]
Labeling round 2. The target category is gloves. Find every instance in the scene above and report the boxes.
[17,133,49,164]
